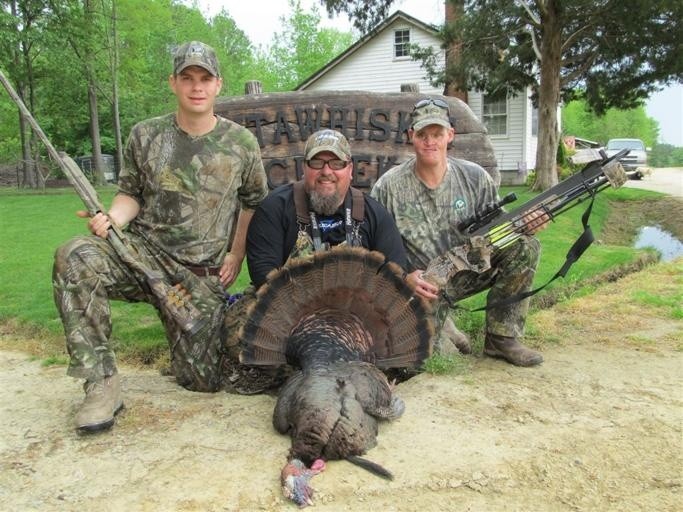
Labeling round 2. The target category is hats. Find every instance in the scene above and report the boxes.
[174,41,218,77]
[304,130,351,161]
[411,102,449,133]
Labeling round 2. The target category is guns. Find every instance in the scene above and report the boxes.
[421,145,632,354]
[1,64,212,335]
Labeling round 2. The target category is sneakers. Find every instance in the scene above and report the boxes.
[444,317,471,354]
[484,333,543,366]
[73,375,124,431]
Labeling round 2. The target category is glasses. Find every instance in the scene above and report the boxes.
[307,158,347,170]
[414,99,450,115]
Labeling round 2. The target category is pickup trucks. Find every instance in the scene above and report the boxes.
[601,136,652,176]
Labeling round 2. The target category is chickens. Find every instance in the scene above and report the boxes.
[220,247,436,508]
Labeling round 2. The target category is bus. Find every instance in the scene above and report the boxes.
[73,154,116,185]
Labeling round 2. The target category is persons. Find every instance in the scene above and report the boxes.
[369,99,544,367]
[51,40,268,433]
[221,128,408,395]
